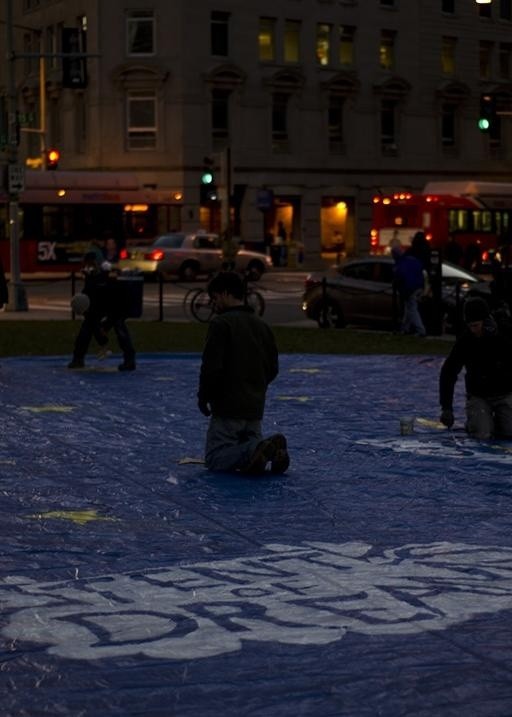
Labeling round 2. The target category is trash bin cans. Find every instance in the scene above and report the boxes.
[123,277,142,317]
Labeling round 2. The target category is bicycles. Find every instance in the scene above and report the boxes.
[189,289,265,322]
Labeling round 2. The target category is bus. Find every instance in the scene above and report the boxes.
[371,191,492,256]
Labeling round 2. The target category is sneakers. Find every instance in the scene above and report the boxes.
[239,432,290,476]
[117,359,135,371]
[67,356,85,370]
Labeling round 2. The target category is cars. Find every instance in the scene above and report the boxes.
[118,232,274,280]
[301,247,467,334]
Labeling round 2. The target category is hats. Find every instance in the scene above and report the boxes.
[463,296,490,323]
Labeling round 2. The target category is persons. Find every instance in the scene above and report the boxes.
[401,231,432,272]
[392,249,430,337]
[67,251,138,373]
[212,229,241,276]
[278,220,286,238]
[438,297,511,441]
[194,272,292,473]
[439,235,510,296]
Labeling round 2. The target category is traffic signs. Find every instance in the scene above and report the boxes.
[7,164,25,192]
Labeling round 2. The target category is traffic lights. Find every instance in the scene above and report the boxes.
[479,97,498,134]
[201,173,218,206]
[45,149,61,169]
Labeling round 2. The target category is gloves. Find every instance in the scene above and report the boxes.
[440,410,455,429]
[196,395,212,418]
